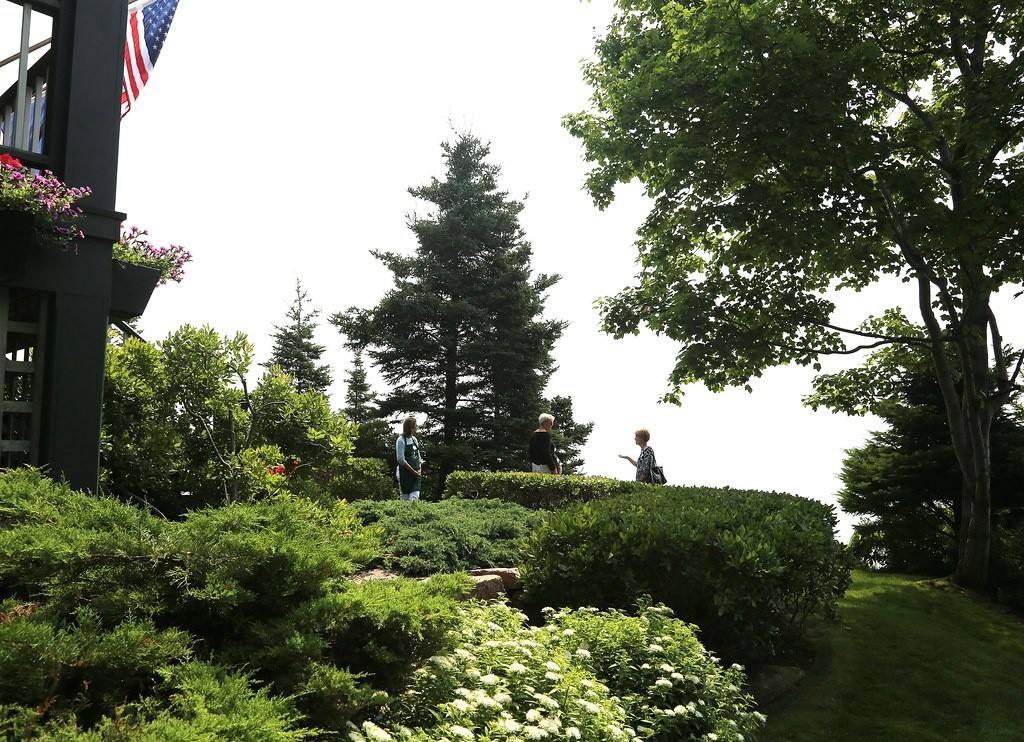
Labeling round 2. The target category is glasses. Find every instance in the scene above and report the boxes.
[547,421,553,426]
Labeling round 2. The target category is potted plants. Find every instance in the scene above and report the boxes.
[0,208,38,252]
[111,260,163,319]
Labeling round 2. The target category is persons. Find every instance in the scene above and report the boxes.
[619,429,655,484]
[396,417,423,500]
[529,413,563,474]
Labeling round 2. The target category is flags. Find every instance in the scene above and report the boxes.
[2,0,180,181]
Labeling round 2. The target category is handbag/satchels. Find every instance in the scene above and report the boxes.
[645,453,667,485]
